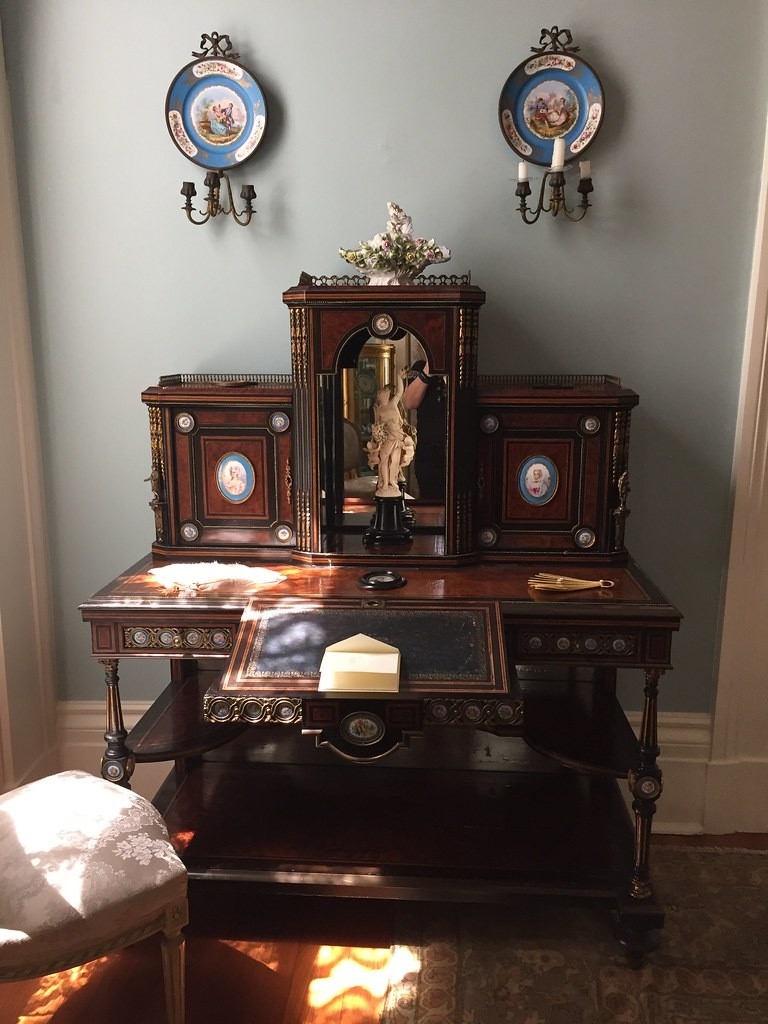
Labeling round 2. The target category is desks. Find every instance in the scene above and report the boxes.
[79,544,680,899]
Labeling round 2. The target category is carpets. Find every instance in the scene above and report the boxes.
[0,829,768,1024]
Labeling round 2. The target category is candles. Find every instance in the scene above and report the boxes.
[578,160,591,179]
[552,135,565,169]
[518,160,528,182]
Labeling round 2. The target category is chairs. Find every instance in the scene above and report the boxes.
[0,772,190,1024]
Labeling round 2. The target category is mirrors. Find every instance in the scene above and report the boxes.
[326,327,448,528]
